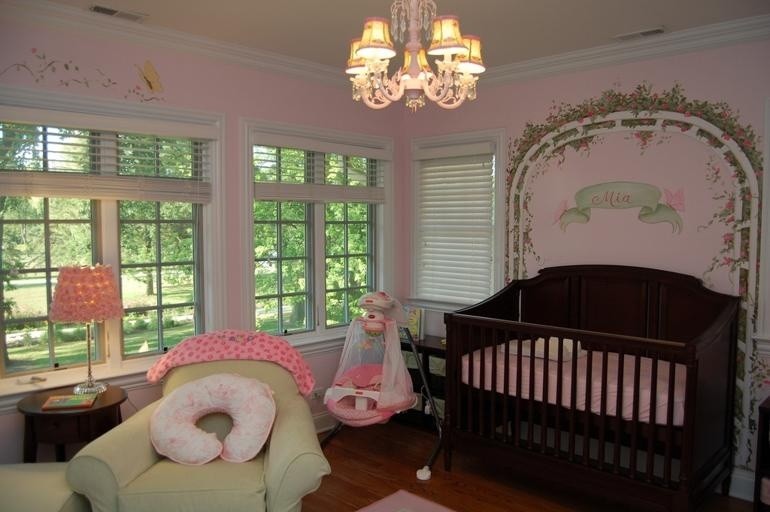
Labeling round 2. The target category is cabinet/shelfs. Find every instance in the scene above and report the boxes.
[390,332,447,435]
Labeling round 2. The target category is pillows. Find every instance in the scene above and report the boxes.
[148,373,275,466]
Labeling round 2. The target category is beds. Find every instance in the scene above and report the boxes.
[444,264,741,512]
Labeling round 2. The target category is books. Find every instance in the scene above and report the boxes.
[41,393,98,411]
[398,305,426,343]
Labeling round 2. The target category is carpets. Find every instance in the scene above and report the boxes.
[356,488,449,512]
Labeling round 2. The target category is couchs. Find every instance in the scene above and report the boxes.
[65,329,332,512]
[4,461,85,512]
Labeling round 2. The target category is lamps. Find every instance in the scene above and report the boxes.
[48,263,125,394]
[345,0,486,112]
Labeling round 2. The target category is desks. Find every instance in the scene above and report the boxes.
[16,384,128,463]
